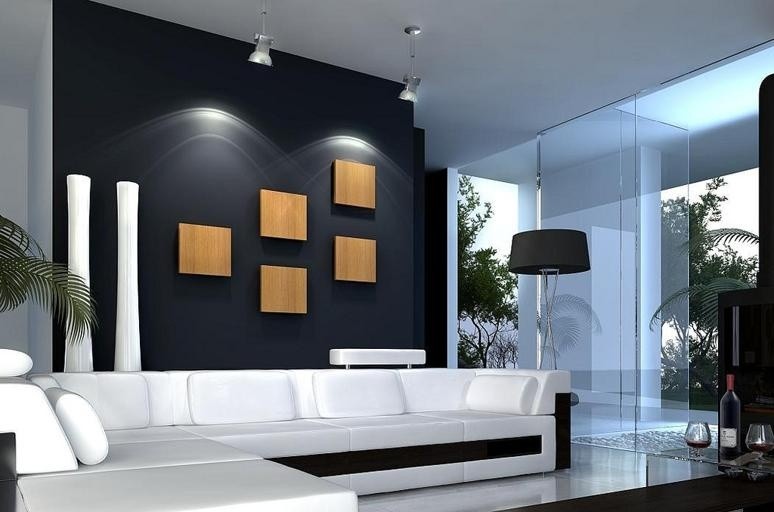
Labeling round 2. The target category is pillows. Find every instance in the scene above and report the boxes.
[467,375,538,416]
[313,371,405,419]
[187,371,295,425]
[0,348,150,464]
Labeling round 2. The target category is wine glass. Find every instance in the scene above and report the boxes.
[684,421,712,463]
[744,421,773,464]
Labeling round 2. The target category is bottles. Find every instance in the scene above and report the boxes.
[718,374,741,470]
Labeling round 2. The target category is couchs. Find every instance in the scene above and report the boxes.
[0,368,571,512]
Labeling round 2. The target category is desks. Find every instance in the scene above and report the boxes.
[496,475,774,512]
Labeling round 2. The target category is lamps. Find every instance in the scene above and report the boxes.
[248,0,274,67]
[510,230,590,405]
[398,27,422,102]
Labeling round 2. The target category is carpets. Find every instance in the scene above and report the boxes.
[570,425,718,464]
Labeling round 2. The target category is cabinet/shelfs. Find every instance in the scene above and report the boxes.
[718,286,774,472]
